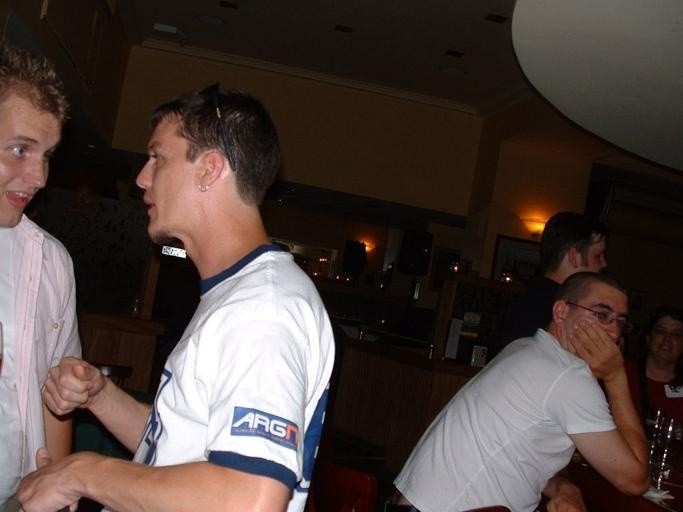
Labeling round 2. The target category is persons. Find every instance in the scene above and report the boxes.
[487,206,607,355]
[386,269,654,512]
[12,86,335,511]
[0,40,79,511]
[582,306,682,512]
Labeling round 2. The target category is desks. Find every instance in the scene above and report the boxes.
[537,424,683,510]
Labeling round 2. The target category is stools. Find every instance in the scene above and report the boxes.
[92,362,133,389]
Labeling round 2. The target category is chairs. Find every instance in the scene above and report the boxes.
[309,463,383,512]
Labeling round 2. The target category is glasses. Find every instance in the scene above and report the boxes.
[565,301,635,335]
[198,79,237,172]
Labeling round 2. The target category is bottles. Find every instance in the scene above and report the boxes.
[644,407,678,494]
